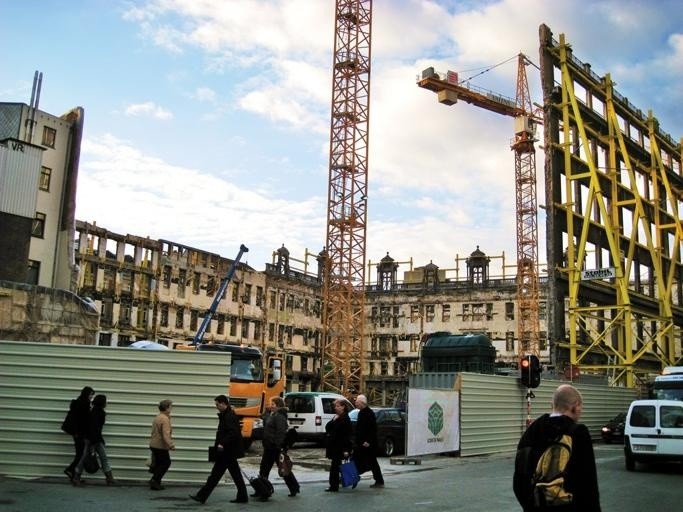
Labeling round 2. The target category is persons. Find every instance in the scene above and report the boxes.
[248,397,300,497]
[71,394,123,485]
[61,385,95,484]
[188,394,249,504]
[510,384,601,511]
[338,393,384,488]
[323,399,361,492]
[146,398,177,491]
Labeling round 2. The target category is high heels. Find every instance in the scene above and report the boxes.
[250,491,258,498]
[286,484,300,497]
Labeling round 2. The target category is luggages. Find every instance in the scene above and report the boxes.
[248,475,275,501]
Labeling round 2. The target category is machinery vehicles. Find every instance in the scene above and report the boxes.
[129,241,288,458]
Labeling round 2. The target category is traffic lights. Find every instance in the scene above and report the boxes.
[529,354,542,388]
[520,355,529,387]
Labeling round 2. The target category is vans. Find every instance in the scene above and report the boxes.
[283,391,357,447]
[622,398,683,473]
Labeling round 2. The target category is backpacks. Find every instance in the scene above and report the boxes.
[527,429,576,512]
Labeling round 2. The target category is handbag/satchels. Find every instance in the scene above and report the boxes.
[206,445,230,466]
[276,455,294,478]
[62,411,82,436]
[338,461,360,487]
[83,453,101,475]
[283,425,299,451]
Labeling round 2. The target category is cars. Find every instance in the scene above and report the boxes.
[348,405,406,458]
[598,411,630,444]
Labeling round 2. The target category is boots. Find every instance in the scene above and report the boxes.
[70,466,85,487]
[105,471,121,487]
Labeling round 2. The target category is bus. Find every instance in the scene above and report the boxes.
[646,365,683,402]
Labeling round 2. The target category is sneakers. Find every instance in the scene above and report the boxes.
[323,486,338,491]
[228,496,248,503]
[64,465,71,476]
[147,477,165,491]
[188,494,205,505]
[350,475,360,489]
[369,480,385,489]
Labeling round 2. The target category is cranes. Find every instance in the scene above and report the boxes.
[410,47,681,370]
